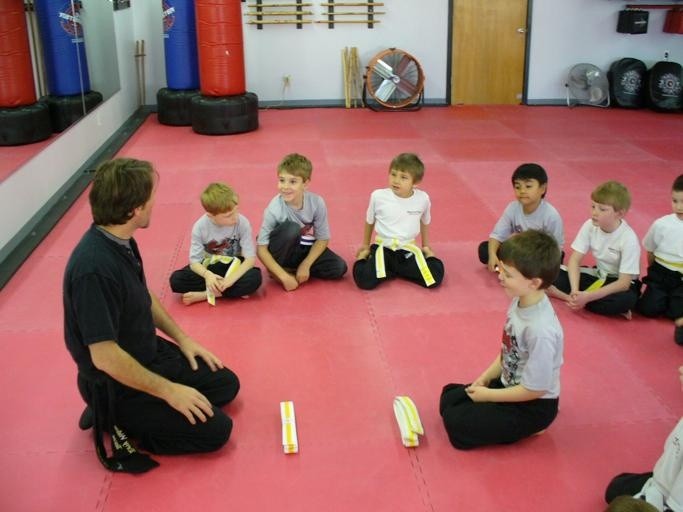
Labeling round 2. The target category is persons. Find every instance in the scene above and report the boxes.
[352,149,444,290]
[603,360,682,511]
[169,181,262,306]
[543,177,643,322]
[62,156,240,473]
[438,223,566,451]
[479,162,566,279]
[255,151,347,291]
[633,173,681,320]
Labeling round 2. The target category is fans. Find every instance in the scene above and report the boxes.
[564,62,612,109]
[362,47,425,112]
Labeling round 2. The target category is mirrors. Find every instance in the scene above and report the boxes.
[0,0,121,185]
